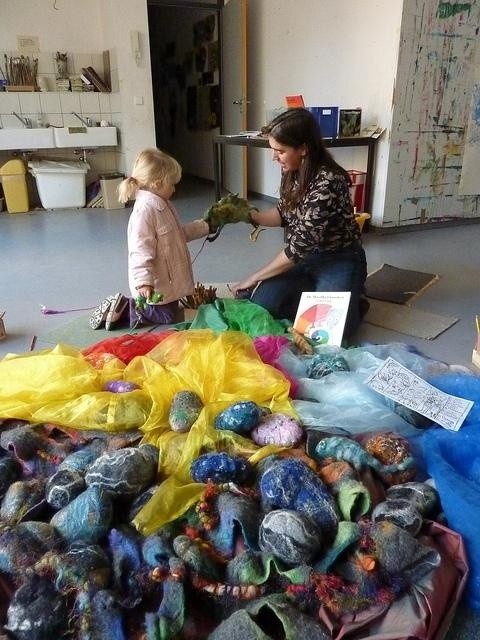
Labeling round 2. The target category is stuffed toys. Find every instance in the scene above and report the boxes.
[135,290,164,308]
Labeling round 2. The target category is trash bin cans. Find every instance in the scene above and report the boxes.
[98,173,126,210]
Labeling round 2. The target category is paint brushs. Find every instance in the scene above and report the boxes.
[0,55,39,85]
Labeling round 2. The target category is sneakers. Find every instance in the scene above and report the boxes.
[90,292,129,331]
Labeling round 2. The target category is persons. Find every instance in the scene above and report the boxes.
[231,108,370,339]
[88,149,220,332]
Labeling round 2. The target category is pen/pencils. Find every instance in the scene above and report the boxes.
[30,336,36,351]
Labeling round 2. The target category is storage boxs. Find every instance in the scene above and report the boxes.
[27,161,90,209]
[305,107,338,139]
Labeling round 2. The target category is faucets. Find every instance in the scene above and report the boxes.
[11,112,32,129]
[72,111,92,127]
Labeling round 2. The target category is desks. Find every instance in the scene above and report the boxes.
[214,134,374,229]
